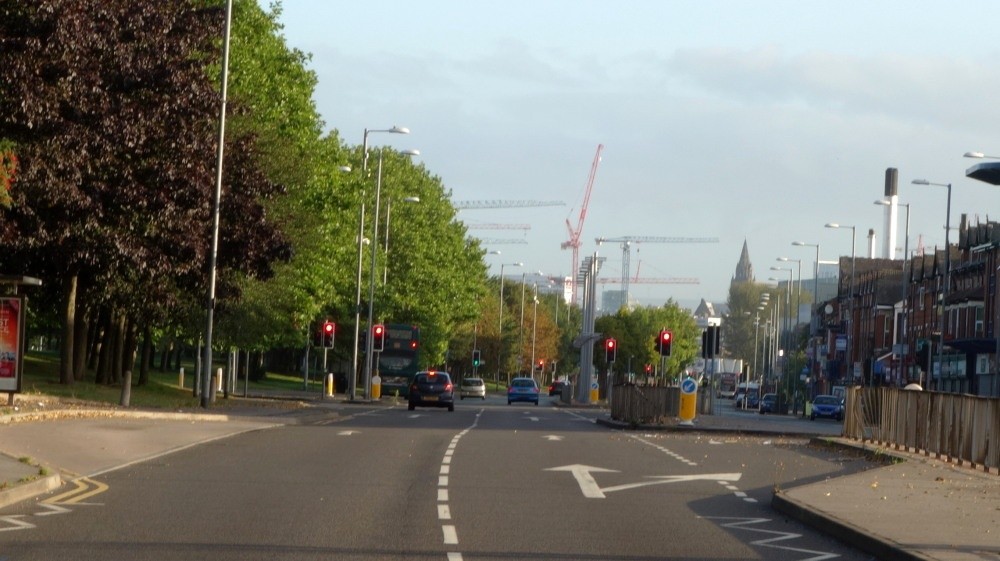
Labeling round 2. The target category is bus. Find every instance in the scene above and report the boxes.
[374,324,418,399]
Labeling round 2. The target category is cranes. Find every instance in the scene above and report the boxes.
[453,198,565,243]
[561,143,604,303]
[595,234,719,307]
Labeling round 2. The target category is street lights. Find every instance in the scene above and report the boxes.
[349,126,420,401]
[476,251,561,378]
[743,151,1000,391]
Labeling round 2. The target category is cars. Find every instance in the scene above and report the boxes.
[759,393,788,415]
[716,381,737,399]
[461,377,485,399]
[549,382,569,396]
[408,371,454,412]
[811,394,842,423]
[508,378,539,405]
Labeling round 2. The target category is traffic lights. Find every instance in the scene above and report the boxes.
[539,361,543,371]
[473,350,480,366]
[372,325,384,352]
[662,332,671,356]
[323,322,334,348]
[654,336,660,352]
[606,339,616,363]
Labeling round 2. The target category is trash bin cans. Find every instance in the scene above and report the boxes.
[335,372,345,394]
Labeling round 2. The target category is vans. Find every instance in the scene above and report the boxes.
[736,383,760,408]
